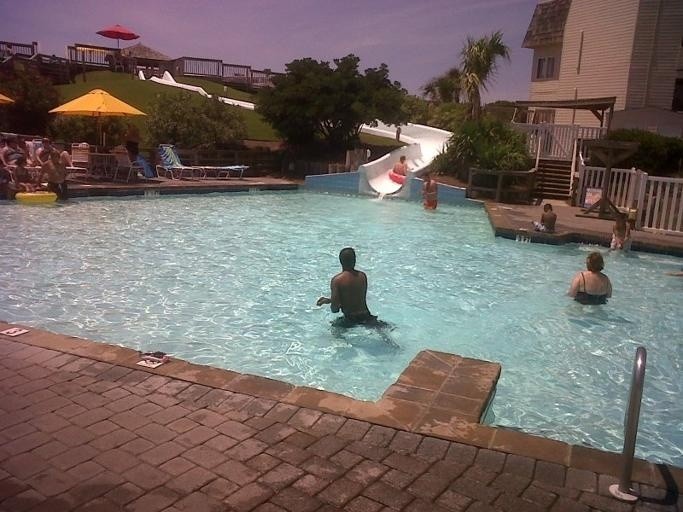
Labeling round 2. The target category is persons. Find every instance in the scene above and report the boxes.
[607,211,634,255]
[391,154,413,176]
[114,49,129,72]
[121,121,142,164]
[419,171,437,211]
[316,246,391,338]
[0,133,76,200]
[530,202,557,233]
[564,250,612,307]
[127,51,137,73]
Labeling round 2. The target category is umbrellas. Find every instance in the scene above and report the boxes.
[1,92,14,104]
[95,22,140,48]
[48,87,147,147]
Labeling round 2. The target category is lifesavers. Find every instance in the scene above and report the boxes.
[16,190,57,201]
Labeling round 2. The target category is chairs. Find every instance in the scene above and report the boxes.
[1,136,249,191]
[293,134,367,175]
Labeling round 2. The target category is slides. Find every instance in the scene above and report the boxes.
[138,70,455,198]
